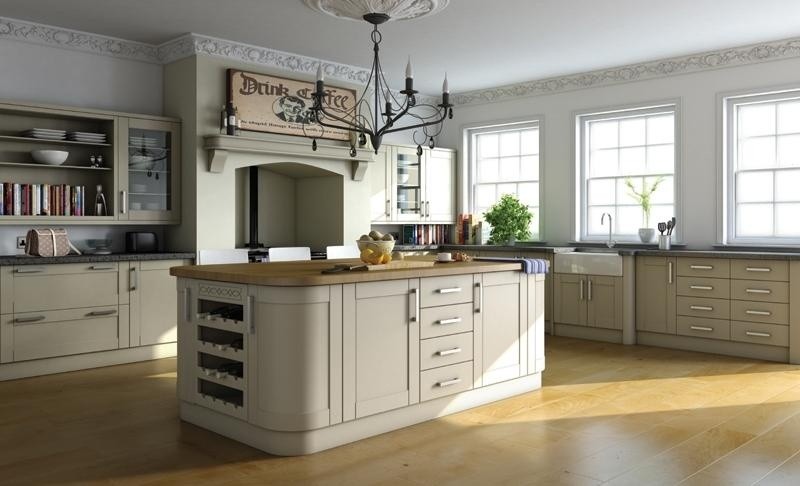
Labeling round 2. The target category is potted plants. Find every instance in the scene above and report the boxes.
[487,195,531,247]
[620,177,660,244]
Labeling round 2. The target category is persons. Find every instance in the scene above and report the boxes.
[277,95,305,123]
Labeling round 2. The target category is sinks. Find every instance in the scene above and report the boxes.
[555,252,622,277]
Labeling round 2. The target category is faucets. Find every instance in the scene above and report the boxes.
[600,211,611,247]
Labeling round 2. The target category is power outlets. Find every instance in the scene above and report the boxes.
[17,236,26,248]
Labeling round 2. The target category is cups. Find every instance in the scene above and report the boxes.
[658,235,670,250]
[437,253,451,260]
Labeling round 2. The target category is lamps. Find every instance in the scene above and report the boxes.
[308,0,453,155]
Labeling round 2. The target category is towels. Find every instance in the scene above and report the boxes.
[473,257,549,274]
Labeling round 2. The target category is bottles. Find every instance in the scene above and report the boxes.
[95,185,104,215]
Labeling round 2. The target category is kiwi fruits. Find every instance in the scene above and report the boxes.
[360,231,394,242]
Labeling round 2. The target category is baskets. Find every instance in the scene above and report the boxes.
[356,240,396,255]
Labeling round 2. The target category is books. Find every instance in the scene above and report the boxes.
[0,182,85,217]
[402,224,451,246]
[458,214,482,245]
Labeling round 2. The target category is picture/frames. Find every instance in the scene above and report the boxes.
[226,68,356,142]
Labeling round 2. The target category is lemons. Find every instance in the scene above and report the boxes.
[360,248,374,263]
[369,252,384,265]
[381,255,392,264]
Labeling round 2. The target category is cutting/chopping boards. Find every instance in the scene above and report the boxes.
[333,260,435,270]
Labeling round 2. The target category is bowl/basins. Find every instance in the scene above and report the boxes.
[30,149,69,165]
[356,240,395,257]
[398,174,409,184]
[129,156,155,171]
[129,202,141,210]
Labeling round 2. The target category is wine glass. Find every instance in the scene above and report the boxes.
[90,156,95,168]
[96,156,102,168]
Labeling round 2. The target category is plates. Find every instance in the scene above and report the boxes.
[129,184,147,191]
[21,129,66,139]
[398,160,411,166]
[434,258,456,262]
[70,132,106,142]
[129,137,159,146]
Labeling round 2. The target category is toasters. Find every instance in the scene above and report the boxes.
[125,231,160,253]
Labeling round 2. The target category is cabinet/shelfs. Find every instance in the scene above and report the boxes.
[129,259,183,347]
[636,256,677,334]
[473,274,518,387]
[185,281,248,425]
[416,274,473,402]
[342,276,420,423]
[0,134,113,220]
[677,258,730,339]
[247,284,329,434]
[555,273,622,330]
[371,145,453,222]
[118,118,177,220]
[13,263,119,362]
[730,258,790,347]
